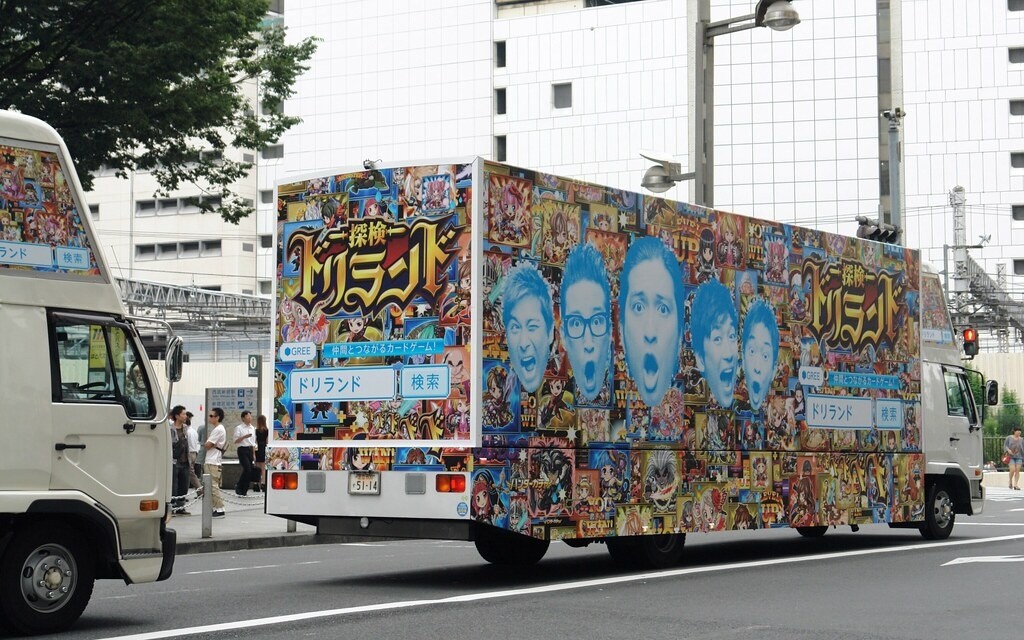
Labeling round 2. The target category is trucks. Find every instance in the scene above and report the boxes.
[266,156,999,567]
[0,110,184,632]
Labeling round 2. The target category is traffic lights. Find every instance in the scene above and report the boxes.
[963,327,979,355]
[860,224,896,241]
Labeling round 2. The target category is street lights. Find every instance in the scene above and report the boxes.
[640,1,802,209]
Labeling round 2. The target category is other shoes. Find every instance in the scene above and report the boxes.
[212,510,225,518]
[185,499,189,502]
[1009,484,1013,489]
[194,494,202,499]
[171,509,191,517]
[1014,486,1020,490]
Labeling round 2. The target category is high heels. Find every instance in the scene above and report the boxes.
[253,484,260,492]
[261,484,266,492]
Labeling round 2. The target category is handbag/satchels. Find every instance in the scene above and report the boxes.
[215,441,229,455]
[249,462,261,482]
[1002,455,1010,465]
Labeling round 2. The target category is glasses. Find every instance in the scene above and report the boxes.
[564,312,609,339]
[210,415,217,418]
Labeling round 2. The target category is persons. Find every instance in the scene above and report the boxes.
[742,302,779,410]
[253,415,268,492]
[689,280,739,408]
[232,411,258,496]
[173,412,205,499]
[1004,428,1024,490]
[560,250,612,401]
[203,408,226,519]
[500,261,554,392]
[170,406,191,517]
[618,239,684,407]
